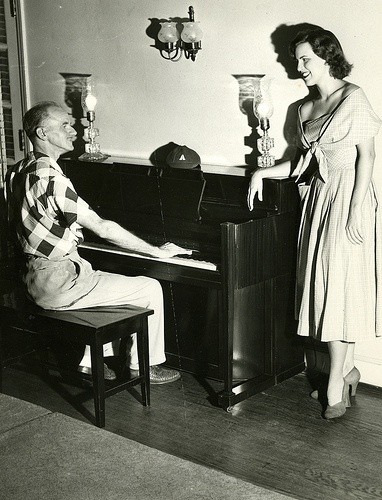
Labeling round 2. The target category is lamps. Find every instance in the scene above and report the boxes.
[158,5,202,62]
[253,76,275,174]
[79,76,109,162]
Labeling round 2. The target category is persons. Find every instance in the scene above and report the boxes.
[246,29,382,419]
[2,101,193,384]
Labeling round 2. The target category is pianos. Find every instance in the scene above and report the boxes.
[56,152,306,414]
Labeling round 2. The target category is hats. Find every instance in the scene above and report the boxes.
[167,146,201,169]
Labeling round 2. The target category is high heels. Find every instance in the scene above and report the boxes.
[311,366,360,399]
[325,377,351,418]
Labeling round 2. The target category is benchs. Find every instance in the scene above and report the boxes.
[0,291,155,427]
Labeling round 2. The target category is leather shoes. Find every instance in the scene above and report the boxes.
[78,362,116,380]
[128,365,181,384]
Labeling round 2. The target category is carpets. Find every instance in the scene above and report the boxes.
[0,392,297,500]
